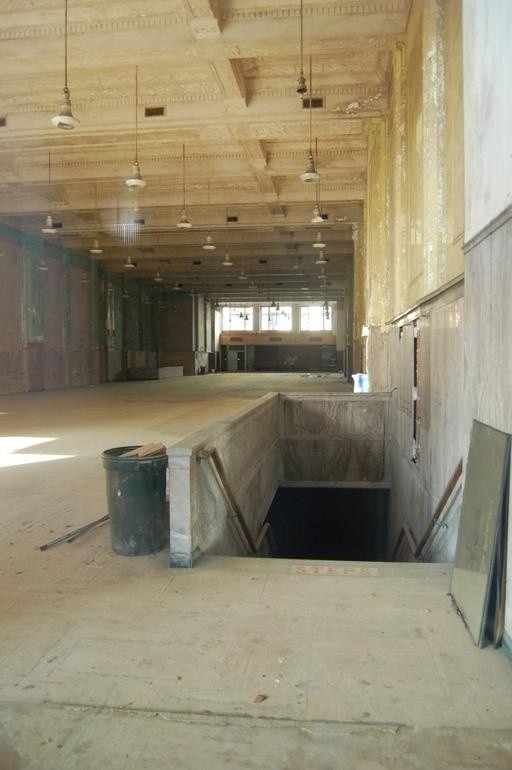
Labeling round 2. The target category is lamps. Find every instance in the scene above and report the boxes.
[123,65,147,192]
[310,137,325,224]
[40,152,59,236]
[175,144,193,230]
[300,54,321,184]
[120,207,332,322]
[88,181,105,257]
[51,2,81,130]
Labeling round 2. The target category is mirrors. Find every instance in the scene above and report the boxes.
[448,417,512,650]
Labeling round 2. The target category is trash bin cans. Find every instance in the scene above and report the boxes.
[101,444,169,559]
[200,367,205,374]
